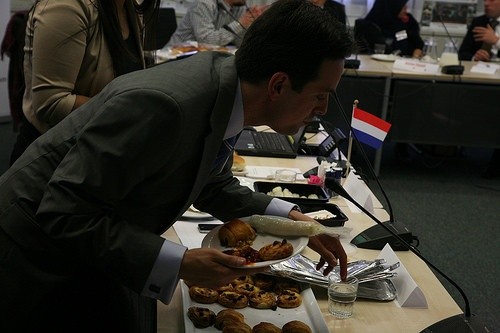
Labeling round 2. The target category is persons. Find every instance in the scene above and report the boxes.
[355,0,425,59]
[0,10,29,165]
[0,1,355,333]
[310,0,350,28]
[458,0,500,62]
[9,1,160,167]
[163,0,273,51]
[132,0,161,52]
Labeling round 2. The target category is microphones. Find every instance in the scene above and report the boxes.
[222,24,242,40]
[217,2,247,31]
[436,16,464,75]
[329,91,412,250]
[325,177,490,333]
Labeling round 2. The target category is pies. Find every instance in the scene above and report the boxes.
[248,214,319,238]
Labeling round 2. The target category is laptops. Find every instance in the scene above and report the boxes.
[234,123,309,159]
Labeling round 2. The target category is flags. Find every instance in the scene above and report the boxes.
[349,104,392,151]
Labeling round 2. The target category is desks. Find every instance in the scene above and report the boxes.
[157,126,474,333]
[340,54,500,176]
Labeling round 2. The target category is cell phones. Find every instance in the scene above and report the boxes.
[198,224,221,233]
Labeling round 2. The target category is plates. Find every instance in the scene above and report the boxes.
[202,215,309,268]
[180,279,329,333]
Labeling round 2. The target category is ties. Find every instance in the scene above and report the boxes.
[482,19,499,51]
[212,134,238,174]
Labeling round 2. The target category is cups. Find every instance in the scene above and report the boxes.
[374,44,385,54]
[328,274,359,318]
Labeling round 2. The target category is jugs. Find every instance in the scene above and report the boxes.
[439,38,459,66]
[419,36,438,64]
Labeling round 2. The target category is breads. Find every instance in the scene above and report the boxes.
[183,273,302,309]
[258,239,294,260]
[187,307,312,333]
[219,219,256,247]
